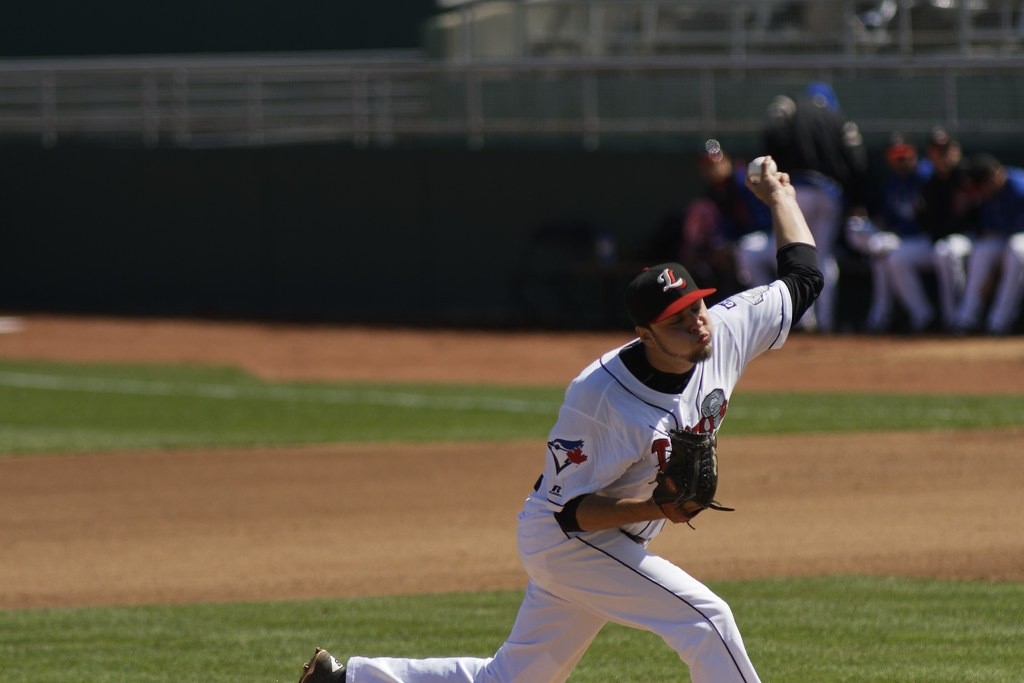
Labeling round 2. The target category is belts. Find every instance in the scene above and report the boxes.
[534,476,645,543]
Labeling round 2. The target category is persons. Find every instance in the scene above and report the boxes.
[686,84,1024,340]
[299,155,826,683]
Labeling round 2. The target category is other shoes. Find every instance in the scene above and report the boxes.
[299,646,345,683]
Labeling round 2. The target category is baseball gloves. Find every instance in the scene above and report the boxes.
[647,423,736,531]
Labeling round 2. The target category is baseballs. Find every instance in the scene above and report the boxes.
[748,155,778,185]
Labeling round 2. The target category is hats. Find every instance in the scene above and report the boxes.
[623,262,717,324]
[885,132,918,160]
